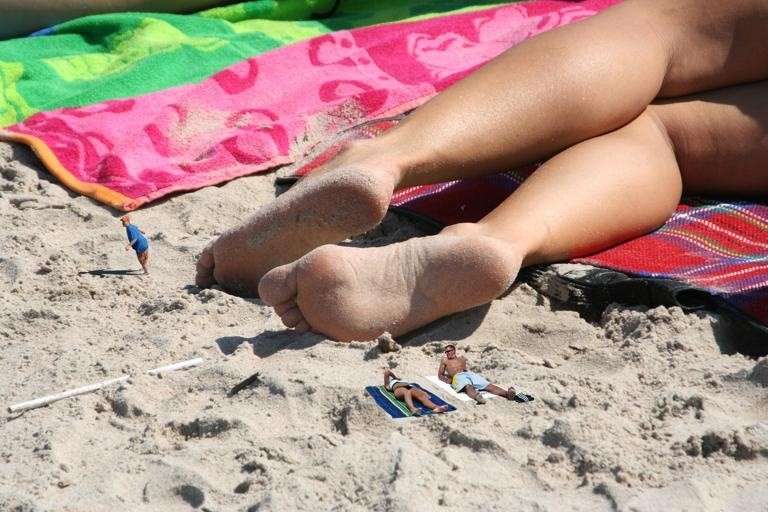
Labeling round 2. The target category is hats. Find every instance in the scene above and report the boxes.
[121,216,130,221]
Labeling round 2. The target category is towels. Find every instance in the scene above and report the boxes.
[0,0,624,214]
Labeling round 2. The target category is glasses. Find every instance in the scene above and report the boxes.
[445,350,452,353]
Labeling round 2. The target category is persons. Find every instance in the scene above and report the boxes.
[436,343,517,405]
[120,215,150,276]
[382,366,450,418]
[191,2,766,346]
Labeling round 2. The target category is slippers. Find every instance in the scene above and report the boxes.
[514,392,531,400]
[517,395,534,403]
